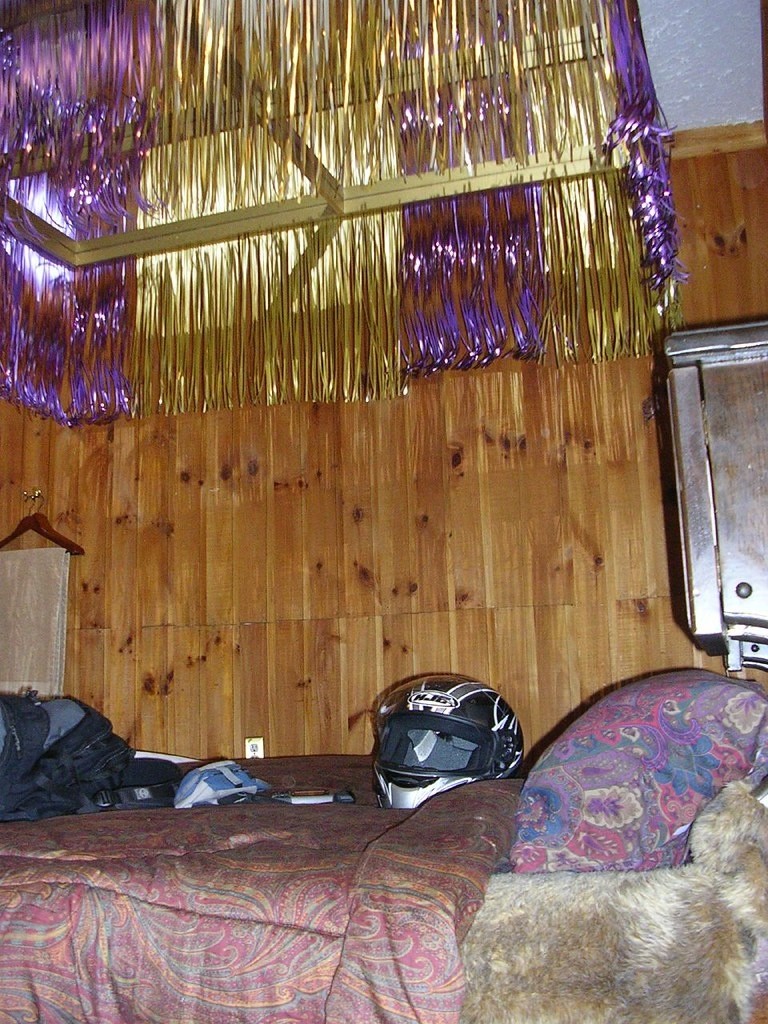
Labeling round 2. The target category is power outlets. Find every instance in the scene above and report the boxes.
[245,738,264,759]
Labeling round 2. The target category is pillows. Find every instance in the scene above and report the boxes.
[510,668,768,871]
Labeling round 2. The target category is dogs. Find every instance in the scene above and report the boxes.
[459,779,768,1024]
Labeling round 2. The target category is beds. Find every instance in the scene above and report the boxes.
[0,753,768,1024]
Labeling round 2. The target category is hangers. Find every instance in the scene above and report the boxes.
[0,493,86,556]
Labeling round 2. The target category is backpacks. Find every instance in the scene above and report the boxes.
[0,688,183,825]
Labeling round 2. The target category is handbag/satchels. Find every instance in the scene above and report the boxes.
[171,759,276,809]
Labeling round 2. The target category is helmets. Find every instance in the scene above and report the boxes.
[372,672,525,809]
